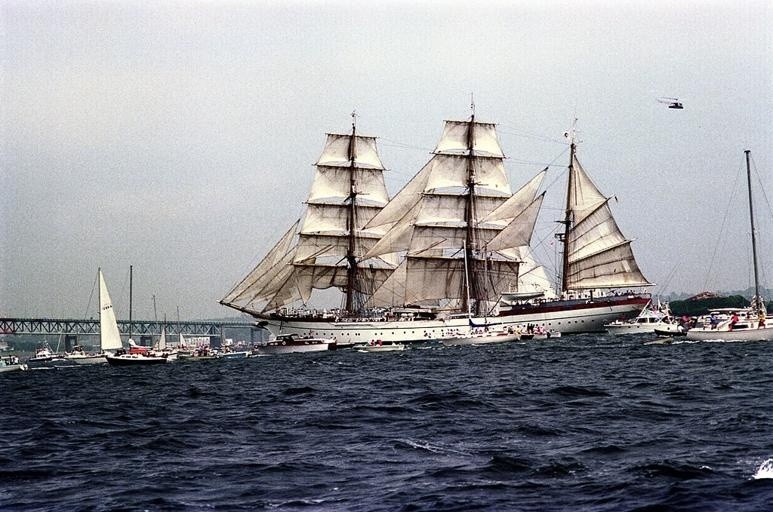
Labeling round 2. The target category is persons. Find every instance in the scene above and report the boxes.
[471,325,544,334]
[371,339,375,346]
[652,306,656,311]
[198,346,203,357]
[626,290,633,295]
[756,310,766,330]
[622,313,625,324]
[710,313,718,330]
[728,312,739,331]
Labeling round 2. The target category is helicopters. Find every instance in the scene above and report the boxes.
[654,95,685,110]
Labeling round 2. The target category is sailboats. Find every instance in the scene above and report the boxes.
[688,148,773,344]
[216,90,657,344]
[64,267,123,367]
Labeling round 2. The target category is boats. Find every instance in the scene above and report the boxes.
[105,329,251,367]
[253,334,339,355]
[600,300,703,337]
[437,326,562,348]
[29,337,64,361]
[0,354,28,372]
[350,339,412,354]
